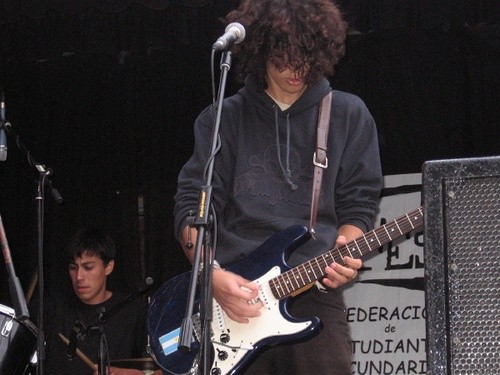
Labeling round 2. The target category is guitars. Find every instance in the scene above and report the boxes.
[146,203,424,375]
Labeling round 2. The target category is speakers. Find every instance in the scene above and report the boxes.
[422,154,500,375]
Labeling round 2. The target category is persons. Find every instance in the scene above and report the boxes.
[173,0,382,375]
[28,227,163,375]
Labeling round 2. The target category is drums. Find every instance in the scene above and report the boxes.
[0,304,45,375]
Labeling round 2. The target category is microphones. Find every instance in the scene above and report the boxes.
[0,99,8,162]
[67,319,82,362]
[214,21,247,51]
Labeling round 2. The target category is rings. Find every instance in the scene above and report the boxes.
[352,270,357,279]
